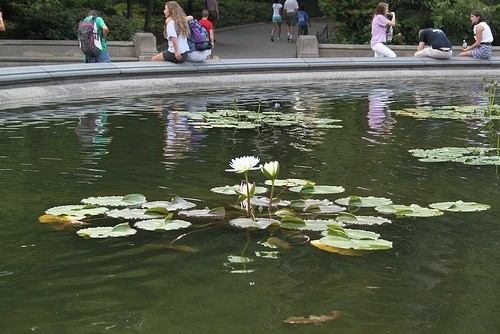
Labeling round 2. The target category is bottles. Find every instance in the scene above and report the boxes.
[387,26,393,42]
[462,40,467,49]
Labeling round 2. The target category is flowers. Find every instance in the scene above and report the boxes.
[225,155,260,218]
[258,160,281,207]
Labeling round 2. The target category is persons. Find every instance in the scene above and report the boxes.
[370,2,397,59]
[151,1,190,63]
[270,0,312,43]
[204,0,220,41]
[188,9,215,62]
[458,9,494,60]
[414,27,454,59]
[0,11,6,31]
[78,10,111,62]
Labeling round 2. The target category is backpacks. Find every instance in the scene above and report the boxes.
[78,16,102,57]
[187,19,213,51]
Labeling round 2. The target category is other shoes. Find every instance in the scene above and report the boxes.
[270,35,274,42]
[287,35,293,42]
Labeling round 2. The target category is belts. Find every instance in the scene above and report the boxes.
[437,48,451,52]
